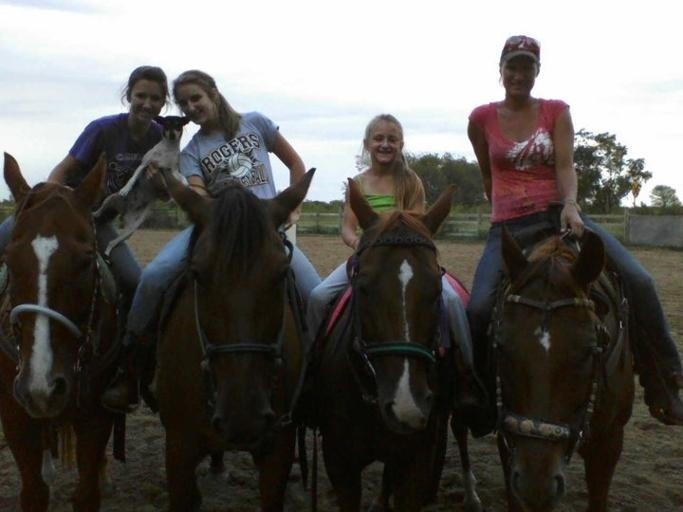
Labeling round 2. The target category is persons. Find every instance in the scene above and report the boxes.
[101,71,322,416]
[306,113,485,413]
[467,34,681,437]
[0,66,168,413]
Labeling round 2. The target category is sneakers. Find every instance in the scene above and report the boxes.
[467,398,498,440]
[643,374,682,425]
[99,384,130,414]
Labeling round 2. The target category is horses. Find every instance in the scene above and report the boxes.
[155,165,309,512]
[0,150,125,512]
[309,178,485,511]
[487,222,635,512]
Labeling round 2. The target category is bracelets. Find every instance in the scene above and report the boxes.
[350,237,359,249]
[562,198,576,206]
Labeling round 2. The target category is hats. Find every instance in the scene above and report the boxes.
[501,35,541,64]
